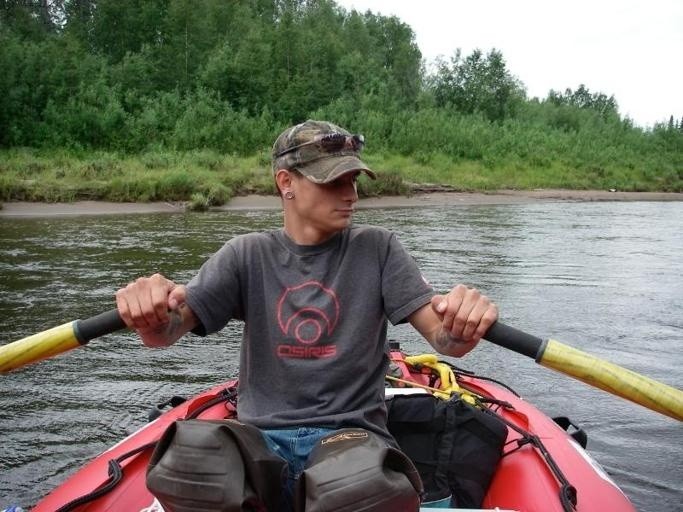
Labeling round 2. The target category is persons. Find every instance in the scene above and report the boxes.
[112,118,499,512]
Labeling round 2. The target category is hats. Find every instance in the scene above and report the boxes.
[271,119,377,186]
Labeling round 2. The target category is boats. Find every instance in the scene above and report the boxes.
[26,335,643,512]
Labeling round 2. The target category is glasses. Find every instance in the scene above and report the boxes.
[276,133,366,157]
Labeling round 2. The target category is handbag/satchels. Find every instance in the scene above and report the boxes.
[384,393,510,509]
